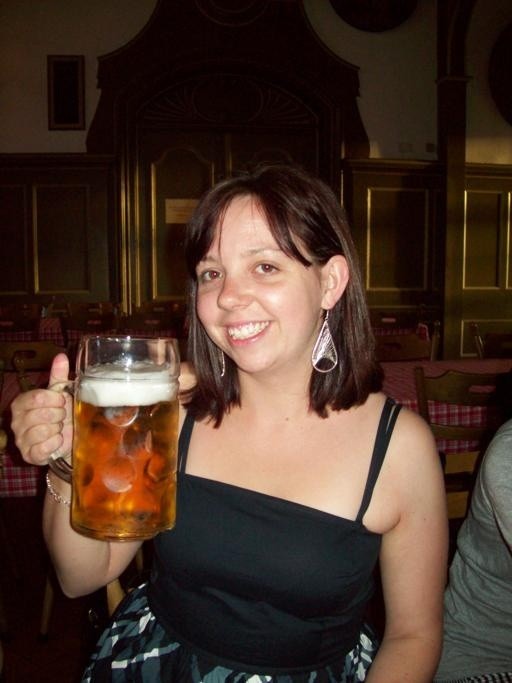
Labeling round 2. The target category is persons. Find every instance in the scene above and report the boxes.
[434,423,512,683]
[8,163,451,682]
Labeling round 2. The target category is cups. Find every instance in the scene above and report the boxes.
[45,333,181,544]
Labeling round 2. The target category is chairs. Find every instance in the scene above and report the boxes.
[372,317,511,510]
[0,301,191,513]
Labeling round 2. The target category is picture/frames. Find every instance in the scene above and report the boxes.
[46,53,87,131]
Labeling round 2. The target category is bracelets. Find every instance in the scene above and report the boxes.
[44,472,72,508]
